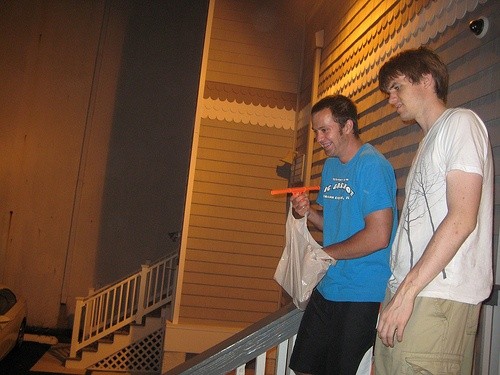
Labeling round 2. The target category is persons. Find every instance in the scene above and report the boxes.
[286,93,400,375]
[369,45,496,375]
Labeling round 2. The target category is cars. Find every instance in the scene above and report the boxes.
[0,284,32,364]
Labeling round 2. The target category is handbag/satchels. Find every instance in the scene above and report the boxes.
[273,201,337,312]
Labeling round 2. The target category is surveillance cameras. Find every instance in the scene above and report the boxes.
[469,15,489,38]
